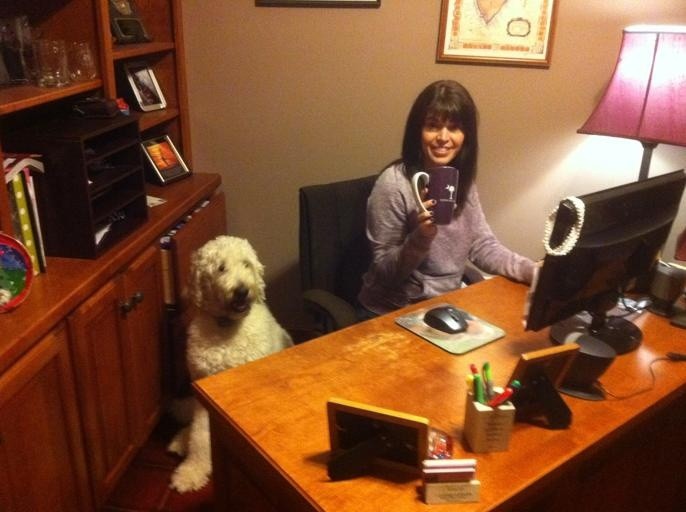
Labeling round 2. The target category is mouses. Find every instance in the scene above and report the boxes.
[425,305,469,333]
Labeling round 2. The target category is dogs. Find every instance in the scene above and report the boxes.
[160,233,296,496]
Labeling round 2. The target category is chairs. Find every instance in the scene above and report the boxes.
[299,174,379,341]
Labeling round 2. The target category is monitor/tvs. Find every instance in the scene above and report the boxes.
[524,170,686,359]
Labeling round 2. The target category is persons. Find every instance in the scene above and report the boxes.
[349,78,549,326]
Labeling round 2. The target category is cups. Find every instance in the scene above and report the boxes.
[410,165,460,226]
[0,13,100,89]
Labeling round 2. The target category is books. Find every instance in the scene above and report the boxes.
[0,145,49,278]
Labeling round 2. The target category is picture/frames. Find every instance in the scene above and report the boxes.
[327,397,430,478]
[505,343,580,423]
[435,0,560,69]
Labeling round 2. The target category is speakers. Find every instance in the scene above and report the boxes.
[646,258,686,318]
[558,334,617,401]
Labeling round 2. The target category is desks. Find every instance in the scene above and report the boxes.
[190,254,686,512]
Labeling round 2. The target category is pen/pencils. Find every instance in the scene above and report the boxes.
[465,362,521,407]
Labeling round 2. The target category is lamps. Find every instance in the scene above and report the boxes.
[576,24,686,184]
